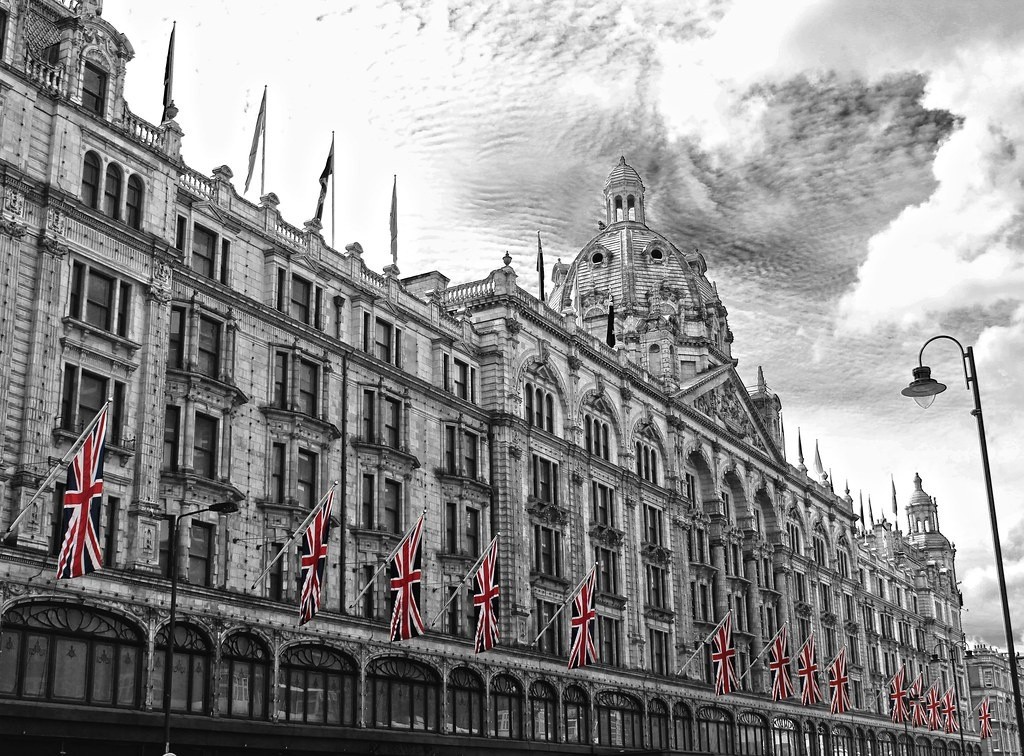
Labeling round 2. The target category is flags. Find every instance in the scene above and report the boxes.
[712,614,740,696]
[390,517,424,642]
[942,687,959,734]
[909,674,929,729]
[890,668,911,723]
[56,409,103,580]
[245,95,265,195]
[926,681,943,731]
[797,634,823,705]
[389,185,397,260]
[568,571,597,669]
[769,626,795,702]
[473,540,500,654]
[160,32,174,123]
[828,649,851,715]
[536,239,545,300]
[978,696,993,739]
[298,490,335,627]
[316,144,333,221]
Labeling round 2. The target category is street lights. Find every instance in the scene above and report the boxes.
[928,641,977,756]
[902,335,1024,756]
[128,500,241,755]
